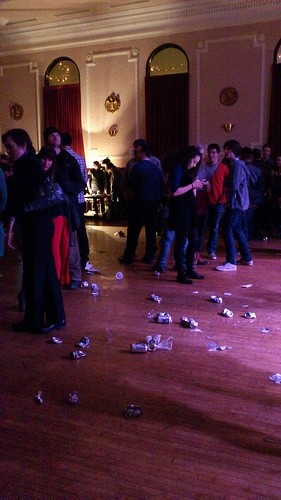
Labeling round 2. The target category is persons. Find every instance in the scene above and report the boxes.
[0,153,15,257]
[44,127,85,289]
[33,146,71,288]
[86,139,280,285]
[61,132,90,271]
[1,128,66,334]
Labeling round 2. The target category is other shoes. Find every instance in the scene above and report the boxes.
[144,254,154,263]
[40,317,66,333]
[152,264,167,272]
[69,281,82,288]
[119,255,133,264]
[12,320,42,334]
[210,253,217,259]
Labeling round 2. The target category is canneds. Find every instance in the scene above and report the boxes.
[91,283,99,296]
[72,350,85,360]
[122,404,143,417]
[243,312,256,319]
[131,334,161,352]
[52,336,63,343]
[80,281,88,287]
[223,308,234,317]
[210,295,223,303]
[78,336,89,348]
[181,317,197,328]
[151,293,163,303]
[157,312,172,323]
[36,391,44,403]
[64,392,79,403]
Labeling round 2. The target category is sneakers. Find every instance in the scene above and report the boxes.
[240,259,254,266]
[189,270,204,280]
[177,273,193,284]
[84,262,101,272]
[216,263,238,271]
